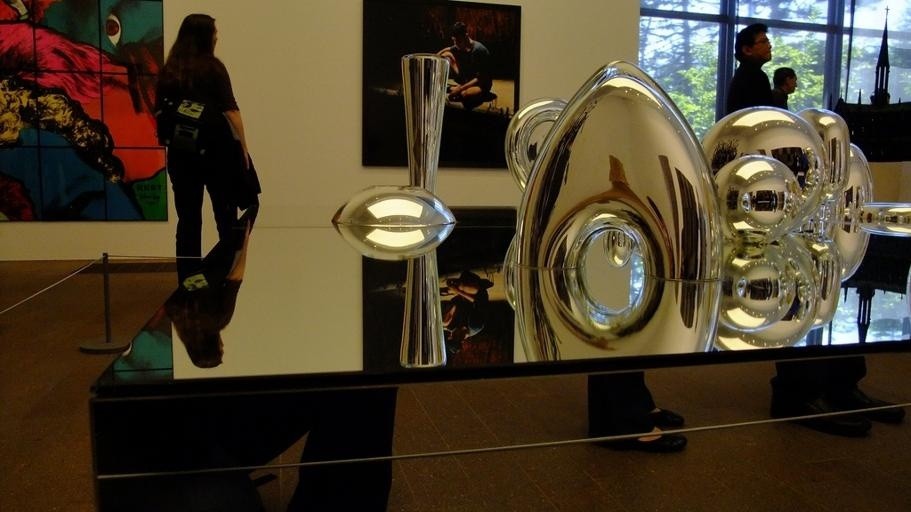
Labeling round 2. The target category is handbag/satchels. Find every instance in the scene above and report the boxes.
[151,83,217,157]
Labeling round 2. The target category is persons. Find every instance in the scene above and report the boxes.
[155,15,262,259]
[438,20,493,99]
[772,67,797,110]
[726,22,772,115]
[441,271,494,353]
[163,204,258,368]
